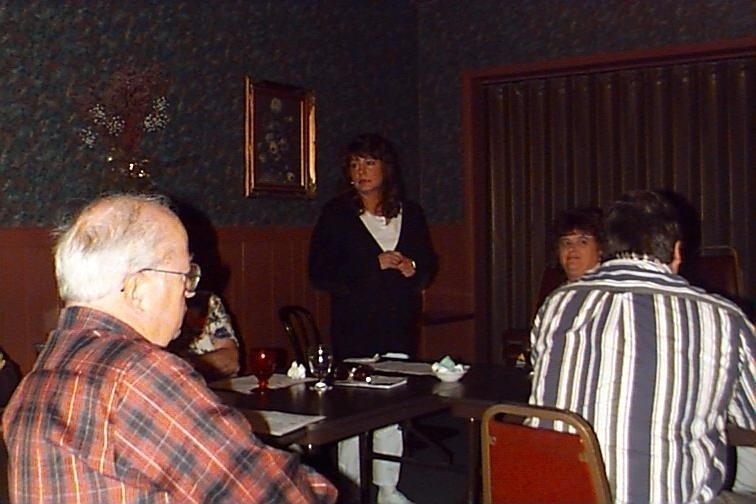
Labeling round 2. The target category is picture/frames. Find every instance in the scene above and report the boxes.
[242,74,320,201]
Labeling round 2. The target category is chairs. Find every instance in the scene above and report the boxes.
[476,399,612,503]
[277,304,322,362]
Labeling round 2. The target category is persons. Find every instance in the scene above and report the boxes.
[0,195,339,503]
[167,292,240,379]
[555,211,604,281]
[524,189,755,503]
[308,134,440,362]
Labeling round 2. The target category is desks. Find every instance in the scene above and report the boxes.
[204,360,531,502]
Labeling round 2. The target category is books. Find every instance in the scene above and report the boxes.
[333,352,434,389]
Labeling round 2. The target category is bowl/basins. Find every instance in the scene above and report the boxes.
[432,369,467,383]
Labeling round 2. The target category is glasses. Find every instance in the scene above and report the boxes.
[119,263,202,300]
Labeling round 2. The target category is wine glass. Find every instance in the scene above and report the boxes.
[251,347,277,397]
[308,343,334,393]
